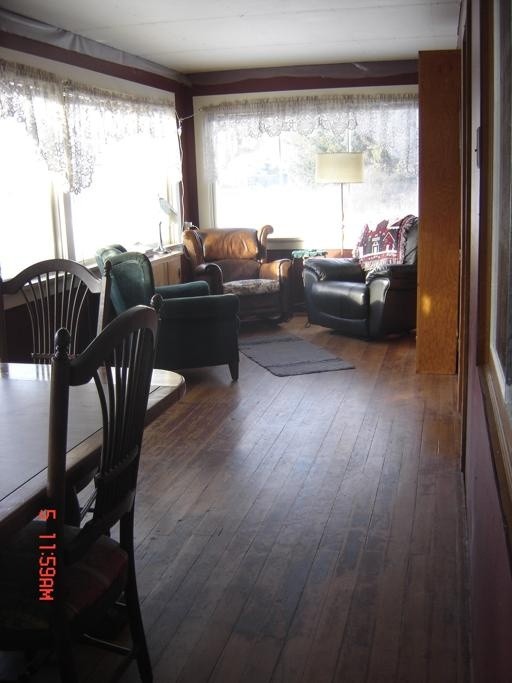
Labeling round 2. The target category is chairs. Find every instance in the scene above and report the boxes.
[303,218,417,342]
[96,244,242,383]
[183,223,293,326]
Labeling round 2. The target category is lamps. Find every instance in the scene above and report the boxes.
[314,151,364,250]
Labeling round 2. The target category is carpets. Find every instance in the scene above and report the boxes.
[238,332,356,376]
